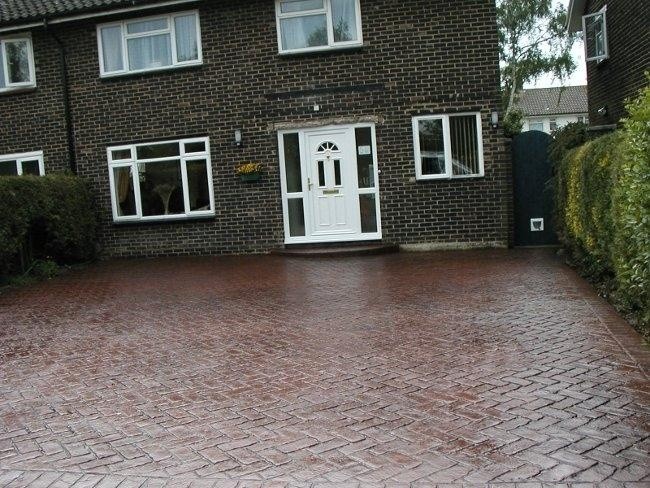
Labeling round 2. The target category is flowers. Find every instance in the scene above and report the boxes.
[234,162,263,173]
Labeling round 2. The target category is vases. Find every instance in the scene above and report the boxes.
[240,174,260,182]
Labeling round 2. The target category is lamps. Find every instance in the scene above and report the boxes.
[235,128,243,147]
[491,111,499,135]
